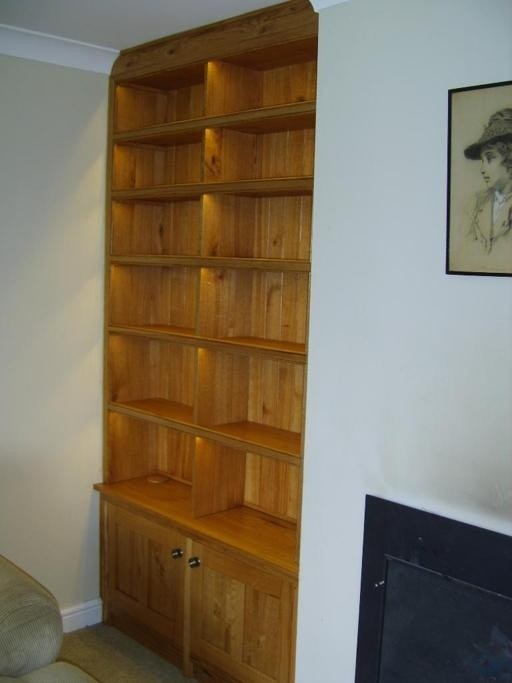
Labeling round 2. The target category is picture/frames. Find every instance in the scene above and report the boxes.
[444,81,511,277]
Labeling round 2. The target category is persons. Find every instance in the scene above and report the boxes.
[464,107,512,255]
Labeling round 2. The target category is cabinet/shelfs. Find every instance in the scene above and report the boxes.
[102,1,320,572]
[93,483,299,682]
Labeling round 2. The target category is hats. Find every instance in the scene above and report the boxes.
[463,107,511,161]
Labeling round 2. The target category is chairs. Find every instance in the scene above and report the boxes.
[1,551,99,681]
